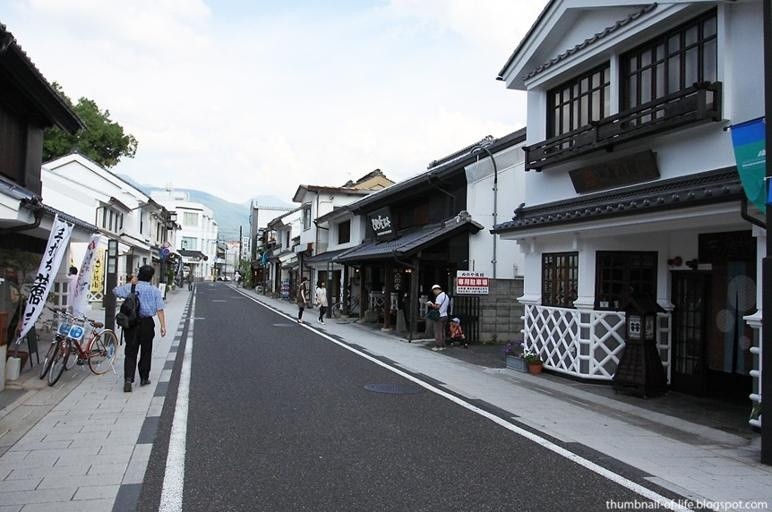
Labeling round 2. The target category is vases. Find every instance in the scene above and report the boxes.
[506,355,528,372]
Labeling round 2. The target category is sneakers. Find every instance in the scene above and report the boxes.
[141,378,151,385]
[432,346,443,351]
[124,382,132,393]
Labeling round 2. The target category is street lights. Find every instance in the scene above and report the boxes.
[181,239,187,255]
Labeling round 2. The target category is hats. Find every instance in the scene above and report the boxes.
[452,318,459,323]
[432,285,440,290]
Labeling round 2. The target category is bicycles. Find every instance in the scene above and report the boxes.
[332,295,361,317]
[289,287,309,303]
[48,307,118,388]
[41,307,86,381]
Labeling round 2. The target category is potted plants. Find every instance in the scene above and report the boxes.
[526,355,543,375]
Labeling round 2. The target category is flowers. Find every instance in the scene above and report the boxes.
[503,339,523,360]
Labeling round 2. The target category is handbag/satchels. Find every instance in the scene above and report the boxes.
[426,309,440,321]
[116,292,140,329]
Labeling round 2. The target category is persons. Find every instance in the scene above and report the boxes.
[316,279,328,325]
[186,270,194,292]
[111,264,166,391]
[449,317,469,348]
[234,271,241,288]
[297,277,308,323]
[426,284,449,352]
[70,267,78,315]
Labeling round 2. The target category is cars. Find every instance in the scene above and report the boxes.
[216,275,231,281]
